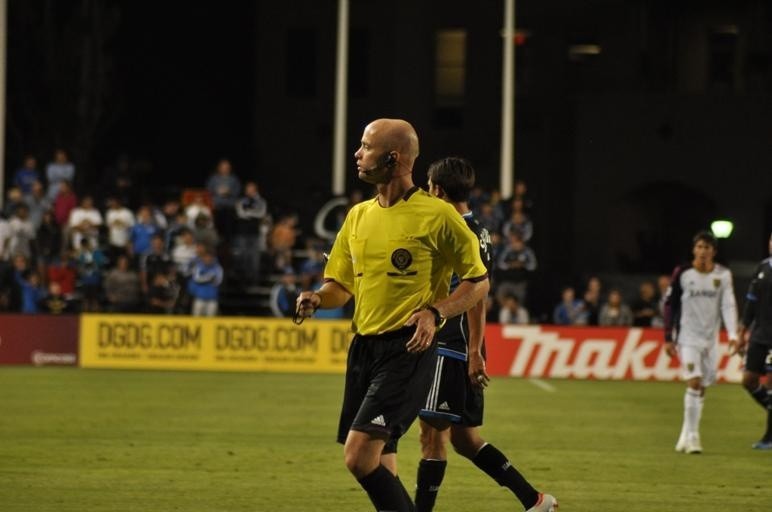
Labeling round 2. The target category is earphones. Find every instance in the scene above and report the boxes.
[387,155,393,164]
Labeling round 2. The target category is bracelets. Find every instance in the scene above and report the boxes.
[425,303,444,326]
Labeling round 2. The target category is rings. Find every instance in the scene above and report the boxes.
[476,375,483,381]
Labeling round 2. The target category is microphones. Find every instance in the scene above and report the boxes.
[363,155,392,177]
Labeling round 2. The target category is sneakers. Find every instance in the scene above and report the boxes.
[675,434,703,453]
[752,437,772,448]
[525,492,558,512]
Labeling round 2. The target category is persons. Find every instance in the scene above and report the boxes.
[661,230,746,455]
[726,232,772,449]
[295,118,490,512]
[408,155,559,511]
[469,180,678,327]
